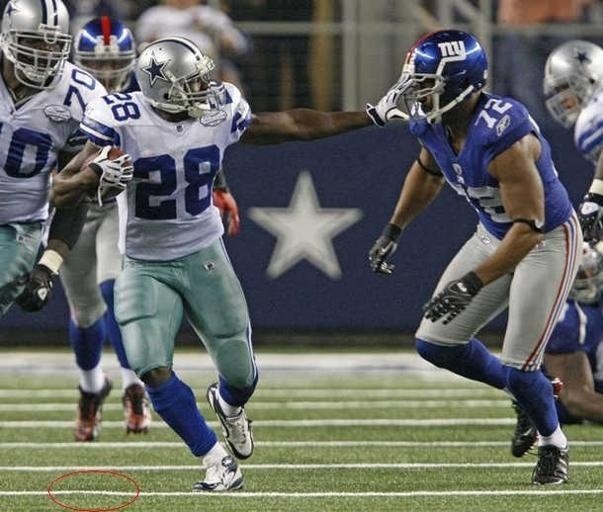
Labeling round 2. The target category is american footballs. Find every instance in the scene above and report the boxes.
[77,147,135,198]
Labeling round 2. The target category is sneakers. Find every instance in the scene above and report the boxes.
[207,382,255,461]
[190,452,244,493]
[73,375,113,444]
[509,365,564,458]
[122,383,149,437]
[531,443,570,488]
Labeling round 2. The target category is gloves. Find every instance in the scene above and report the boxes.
[577,178,603,235]
[367,223,403,278]
[89,144,135,191]
[418,269,485,325]
[365,70,415,129]
[213,186,240,239]
[15,262,60,313]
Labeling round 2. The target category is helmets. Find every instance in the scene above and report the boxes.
[403,29,488,118]
[135,35,211,116]
[541,39,603,130]
[72,16,137,94]
[0,0,70,90]
[568,242,603,305]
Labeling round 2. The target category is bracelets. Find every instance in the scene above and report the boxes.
[39,251,62,277]
[514,217,543,232]
[414,155,445,179]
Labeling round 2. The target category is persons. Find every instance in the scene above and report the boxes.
[41,17,151,440]
[50,34,414,492]
[544,39,602,428]
[135,0,247,89]
[369,28,582,486]
[0,0,108,312]
